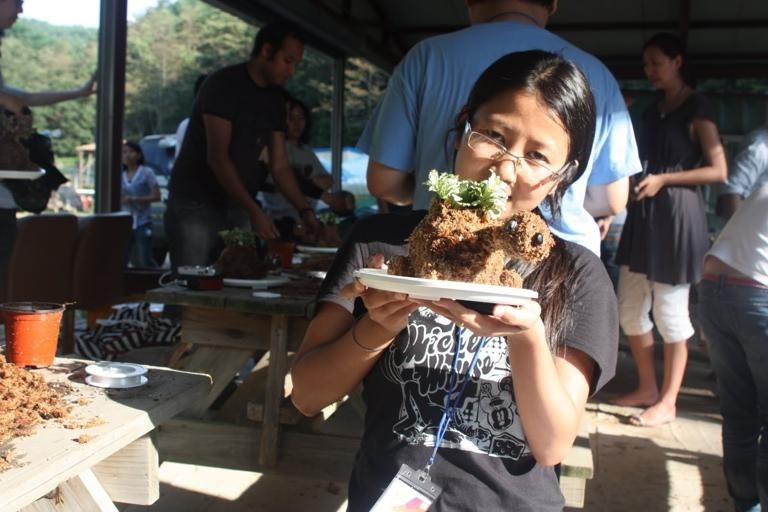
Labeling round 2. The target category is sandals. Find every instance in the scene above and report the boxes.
[625,407,676,427]
[608,393,659,406]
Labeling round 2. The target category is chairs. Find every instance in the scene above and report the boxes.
[0,214,77,318]
[74,212,134,314]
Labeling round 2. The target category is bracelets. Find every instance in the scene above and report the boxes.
[351,323,382,353]
[299,208,314,217]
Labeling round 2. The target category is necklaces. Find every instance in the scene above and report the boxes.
[660,87,684,119]
[486,11,539,25]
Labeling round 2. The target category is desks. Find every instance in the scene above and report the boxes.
[145,256,367,471]
[1,354,213,511]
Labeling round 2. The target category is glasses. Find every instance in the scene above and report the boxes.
[464,120,574,184]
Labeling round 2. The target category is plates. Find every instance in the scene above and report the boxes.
[353,265,543,309]
[0,168,46,182]
[298,242,342,255]
[226,274,292,291]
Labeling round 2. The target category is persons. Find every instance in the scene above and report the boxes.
[120,141,161,268]
[598,31,729,428]
[289,48,619,512]
[0,0,98,267]
[713,125,768,244]
[259,100,333,225]
[697,182,768,512]
[354,0,643,259]
[175,75,208,159]
[163,23,327,281]
